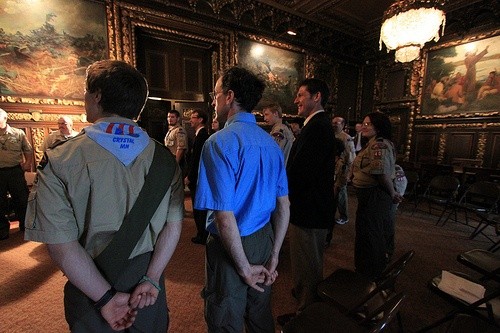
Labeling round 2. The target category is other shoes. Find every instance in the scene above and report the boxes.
[19,224,25,231]
[336,217,350,225]
[0,231,10,239]
[191,237,206,245]
[277,312,297,327]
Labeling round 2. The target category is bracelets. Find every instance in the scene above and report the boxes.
[94,286,117,311]
[139,276,162,292]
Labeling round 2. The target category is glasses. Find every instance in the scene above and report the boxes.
[208,90,223,100]
[58,122,68,126]
[191,117,198,119]
[362,122,372,127]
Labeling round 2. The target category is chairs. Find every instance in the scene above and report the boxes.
[280,249,415,333]
[401,171,500,333]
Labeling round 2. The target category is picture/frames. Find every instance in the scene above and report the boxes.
[229,26,316,120]
[0,0,117,106]
[414,28,500,120]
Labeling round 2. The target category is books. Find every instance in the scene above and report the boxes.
[437,270,487,309]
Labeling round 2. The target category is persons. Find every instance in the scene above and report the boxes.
[330,113,357,225]
[24,58,185,333]
[276,77,336,325]
[163,110,190,218]
[418,135,453,194]
[261,105,301,171]
[0,108,34,240]
[351,122,369,154]
[349,111,403,283]
[191,66,290,333]
[34,116,81,185]
[185,106,224,245]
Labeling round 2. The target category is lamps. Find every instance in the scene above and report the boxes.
[378,0,446,63]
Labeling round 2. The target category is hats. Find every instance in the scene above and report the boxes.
[0,109,8,129]
